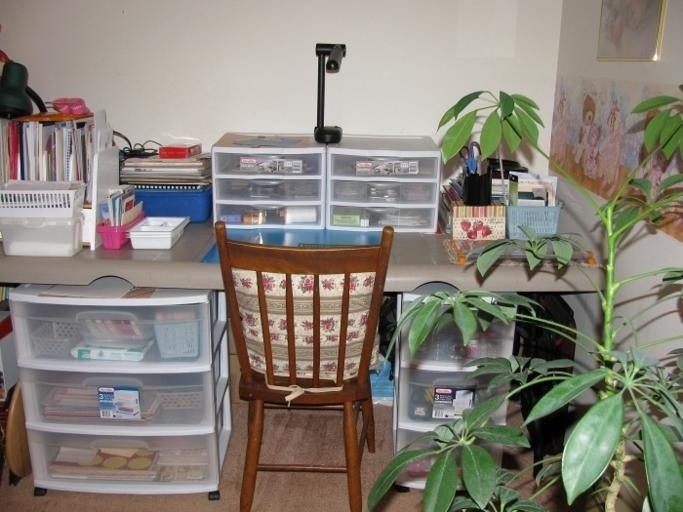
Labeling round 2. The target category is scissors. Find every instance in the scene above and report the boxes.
[459,142,481,170]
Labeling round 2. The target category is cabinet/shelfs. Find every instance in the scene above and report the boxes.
[325,136,443,233]
[7,283,234,501]
[391,292,517,491]
[211,132,327,229]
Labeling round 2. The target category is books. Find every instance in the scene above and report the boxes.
[41,386,162,482]
[0,113,212,209]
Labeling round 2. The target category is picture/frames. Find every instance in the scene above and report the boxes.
[597,0,668,62]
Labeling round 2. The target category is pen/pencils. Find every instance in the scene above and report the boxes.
[464,158,477,175]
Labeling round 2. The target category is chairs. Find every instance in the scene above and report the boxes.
[215,222,393,512]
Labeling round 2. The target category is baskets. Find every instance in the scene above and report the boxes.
[152,319,200,359]
[0,183,85,217]
[505,205,561,240]
[156,389,204,410]
[28,322,84,359]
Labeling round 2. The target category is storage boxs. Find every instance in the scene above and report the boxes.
[134,188,211,222]
[0,213,84,257]
